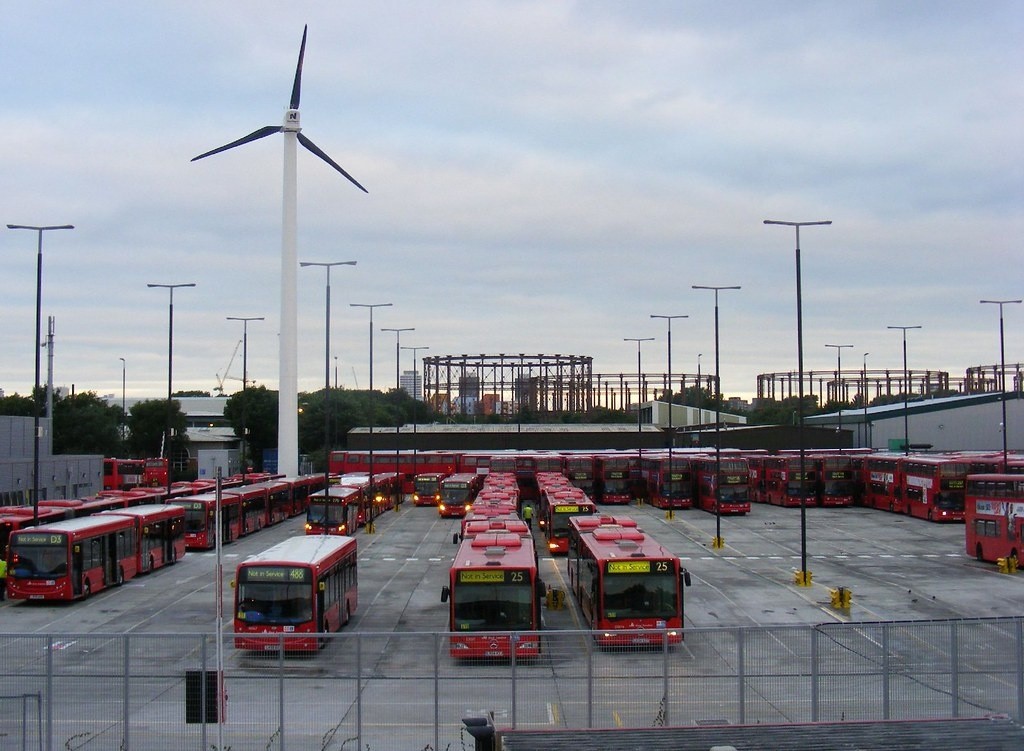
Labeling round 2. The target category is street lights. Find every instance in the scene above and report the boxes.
[823,345,853,454]
[622,313,690,518]
[6,222,75,527]
[351,304,393,527]
[691,284,741,547]
[979,300,1022,472]
[762,219,834,585]
[224,317,265,485]
[401,346,430,476]
[380,327,416,510]
[146,282,197,500]
[887,325,923,454]
[297,260,357,538]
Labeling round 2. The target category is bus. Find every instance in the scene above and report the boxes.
[0,446,1024,580]
[231,535,359,651]
[5,515,137,603]
[566,516,692,648]
[441,521,547,659]
